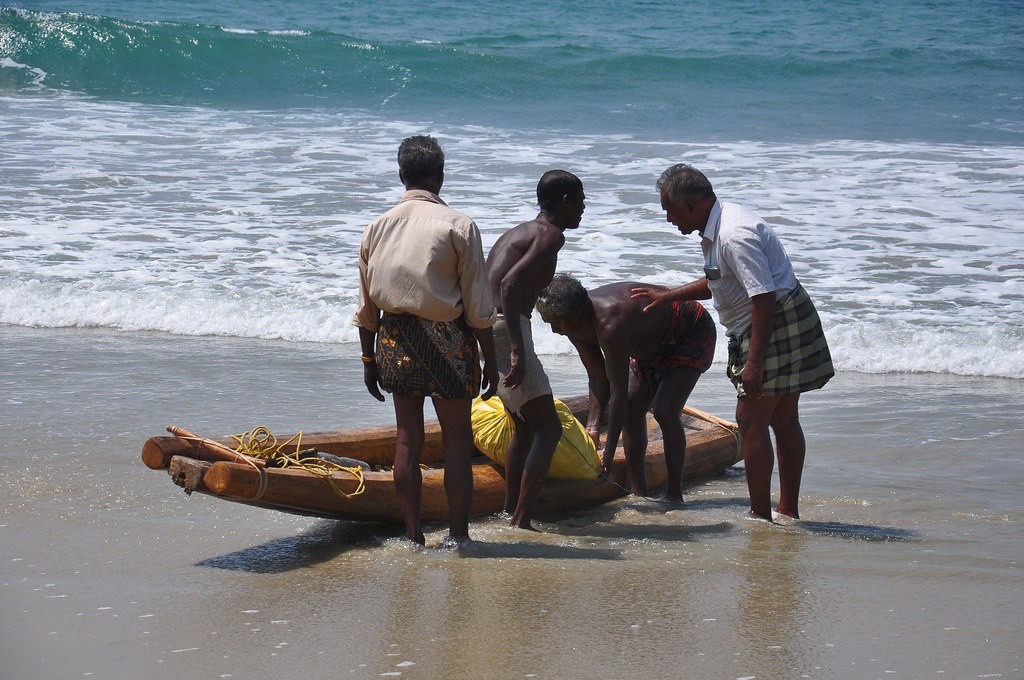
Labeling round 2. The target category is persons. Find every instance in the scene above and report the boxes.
[476,169,587,533]
[628,163,836,519]
[353,135,499,549]
[535,276,718,506]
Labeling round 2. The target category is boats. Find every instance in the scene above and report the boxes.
[140,394,744,526]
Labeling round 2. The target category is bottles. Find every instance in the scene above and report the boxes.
[316,451,371,471]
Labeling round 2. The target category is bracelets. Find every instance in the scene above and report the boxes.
[361,354,376,363]
